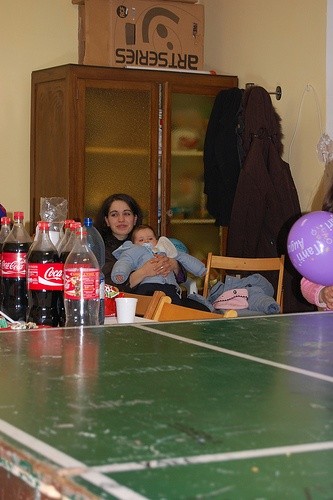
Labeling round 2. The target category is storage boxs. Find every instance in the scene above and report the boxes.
[72,0,204,72]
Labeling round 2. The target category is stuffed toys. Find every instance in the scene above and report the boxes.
[188,273,280,317]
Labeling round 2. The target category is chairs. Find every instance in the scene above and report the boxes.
[203,252,285,315]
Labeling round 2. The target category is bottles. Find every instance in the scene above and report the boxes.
[0,212,105,329]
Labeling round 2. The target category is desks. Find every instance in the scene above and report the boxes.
[0,310,333,500]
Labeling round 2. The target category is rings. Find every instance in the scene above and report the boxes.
[160,266,163,269]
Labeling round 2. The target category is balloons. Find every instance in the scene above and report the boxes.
[286,210,333,286]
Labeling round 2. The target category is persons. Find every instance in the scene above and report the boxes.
[93,194,210,312]
[111,224,206,305]
[300,194,333,312]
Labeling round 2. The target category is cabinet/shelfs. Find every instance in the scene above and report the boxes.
[30,64,239,296]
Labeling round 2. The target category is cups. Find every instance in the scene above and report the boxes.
[115,297,138,323]
